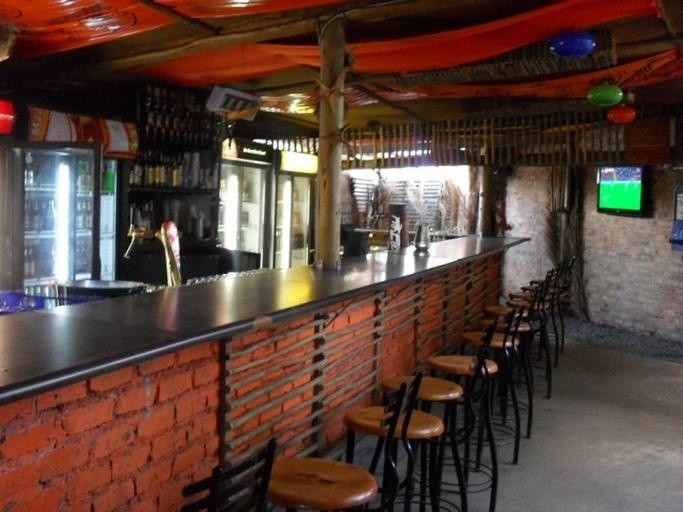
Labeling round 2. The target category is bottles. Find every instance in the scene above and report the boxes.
[142,77,225,146]
[24,154,94,279]
[127,146,223,191]
[128,195,218,242]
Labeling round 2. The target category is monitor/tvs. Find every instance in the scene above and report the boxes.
[595,163,650,217]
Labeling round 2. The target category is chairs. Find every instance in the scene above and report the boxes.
[210,438,277,512]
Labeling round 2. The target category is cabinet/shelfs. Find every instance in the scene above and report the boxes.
[123,85,223,283]
